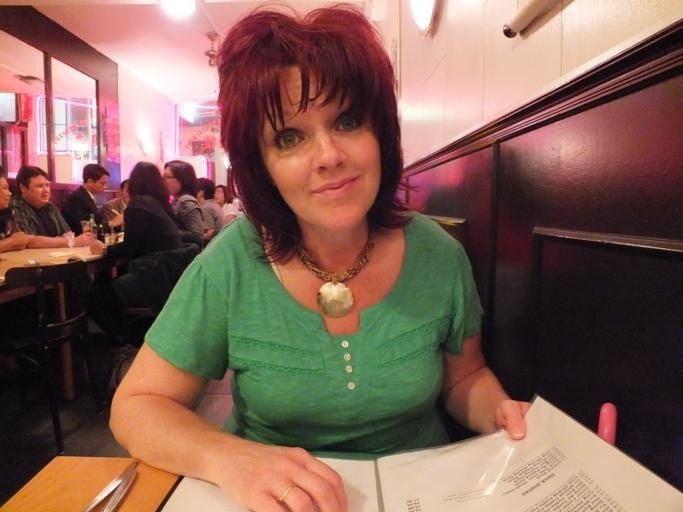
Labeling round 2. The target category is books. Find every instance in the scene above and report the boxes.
[158,387,683,511]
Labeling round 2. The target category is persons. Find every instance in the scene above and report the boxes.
[63,163,131,241]
[0,167,95,254]
[163,161,242,241]
[107,3,532,512]
[78,163,180,350]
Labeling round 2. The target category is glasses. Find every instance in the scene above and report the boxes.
[161,173,176,180]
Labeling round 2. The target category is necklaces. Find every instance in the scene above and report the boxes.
[292,230,376,318]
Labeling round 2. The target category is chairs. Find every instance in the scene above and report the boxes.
[113,241,201,349]
[0,261,107,454]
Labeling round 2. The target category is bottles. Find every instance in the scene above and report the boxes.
[96,221,118,248]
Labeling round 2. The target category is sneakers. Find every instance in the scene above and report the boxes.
[96,365,119,417]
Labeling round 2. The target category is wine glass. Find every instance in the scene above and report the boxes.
[64,230,76,255]
